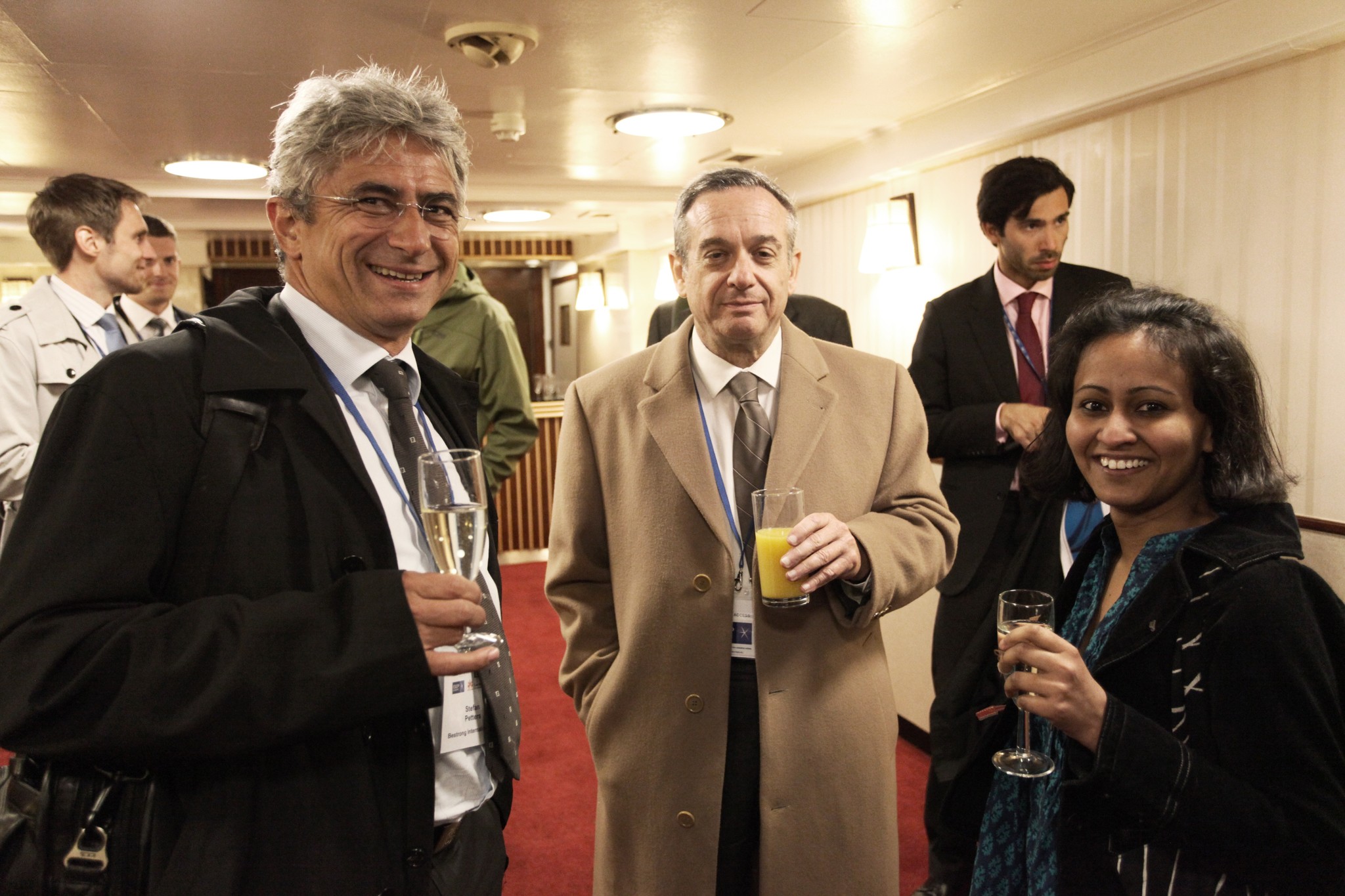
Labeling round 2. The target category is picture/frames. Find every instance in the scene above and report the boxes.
[551,273,580,398]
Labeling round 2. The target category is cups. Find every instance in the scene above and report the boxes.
[752,488,810,607]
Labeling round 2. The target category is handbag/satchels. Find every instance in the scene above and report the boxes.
[0,755,155,895]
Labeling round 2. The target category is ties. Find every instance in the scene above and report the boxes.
[146,318,168,337]
[97,313,129,353]
[367,358,523,785]
[729,371,772,578]
[1015,292,1045,408]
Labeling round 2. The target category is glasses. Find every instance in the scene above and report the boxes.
[305,184,476,239]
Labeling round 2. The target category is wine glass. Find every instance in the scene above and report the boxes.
[992,589,1055,777]
[417,447,504,652]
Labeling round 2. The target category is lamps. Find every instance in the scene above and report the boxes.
[441,22,540,73]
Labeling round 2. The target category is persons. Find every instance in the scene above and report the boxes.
[111,212,200,347]
[542,166,972,895]
[1,170,156,561]
[1,49,528,894]
[646,294,854,348]
[907,152,1135,891]
[968,288,1345,893]
[410,258,542,628]
